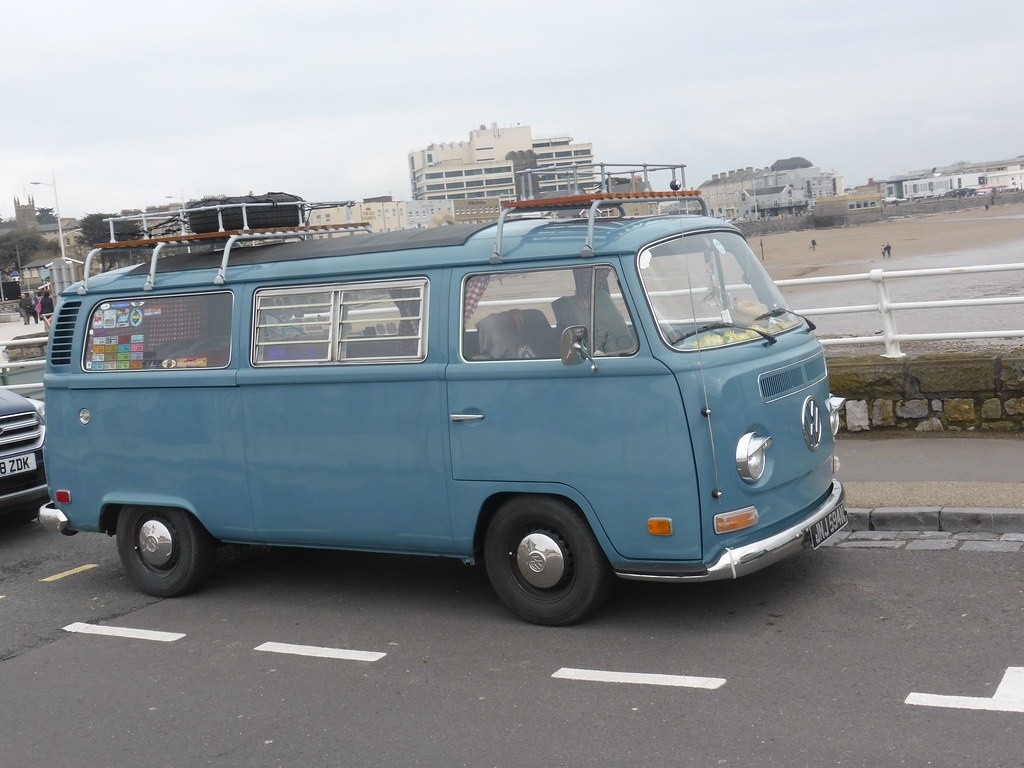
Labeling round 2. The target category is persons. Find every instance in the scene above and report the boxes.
[27,290,40,324]
[881,244,886,257]
[39,290,54,332]
[885,243,891,256]
[19,294,30,326]
[812,239,816,250]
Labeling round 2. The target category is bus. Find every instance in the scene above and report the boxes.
[35,177,855,624]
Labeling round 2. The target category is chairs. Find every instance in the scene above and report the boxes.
[473,288,632,364]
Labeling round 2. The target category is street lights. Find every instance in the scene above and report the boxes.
[165,193,186,215]
[30,174,66,261]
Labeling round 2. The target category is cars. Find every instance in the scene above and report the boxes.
[0,387,47,526]
[881,185,992,207]
